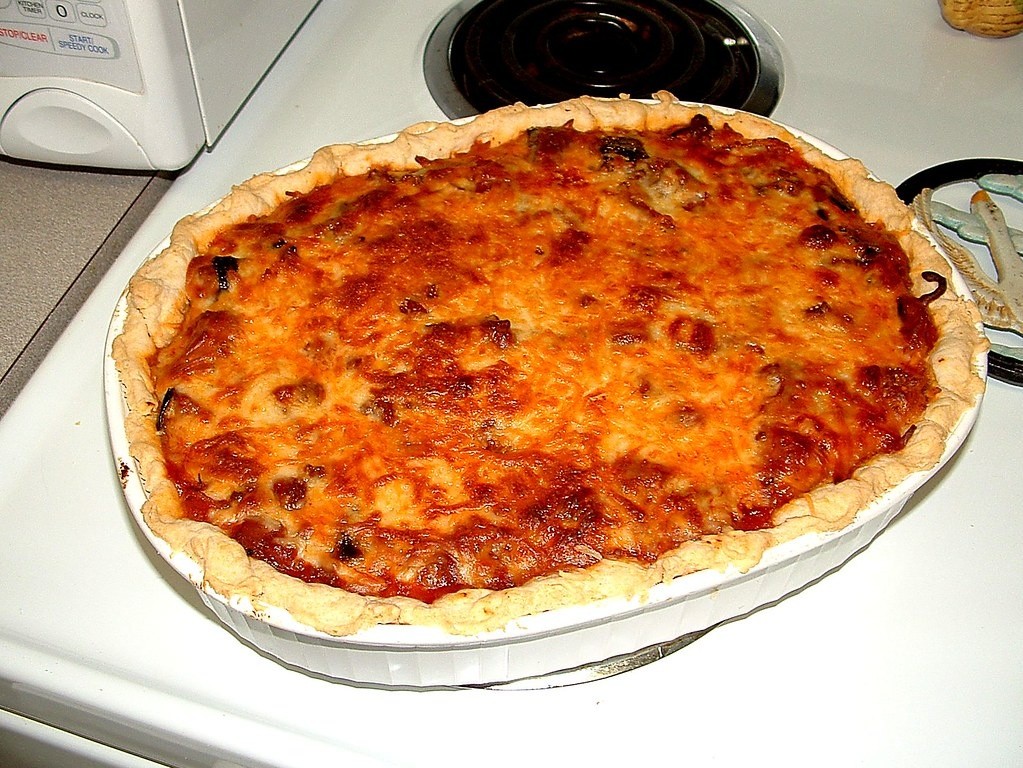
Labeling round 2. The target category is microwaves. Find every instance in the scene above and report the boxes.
[1,1,320,173]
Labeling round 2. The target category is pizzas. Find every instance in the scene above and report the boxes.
[112,88,981,631]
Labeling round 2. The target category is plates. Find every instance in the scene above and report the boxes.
[893,156,1023,387]
[104,99,988,687]
